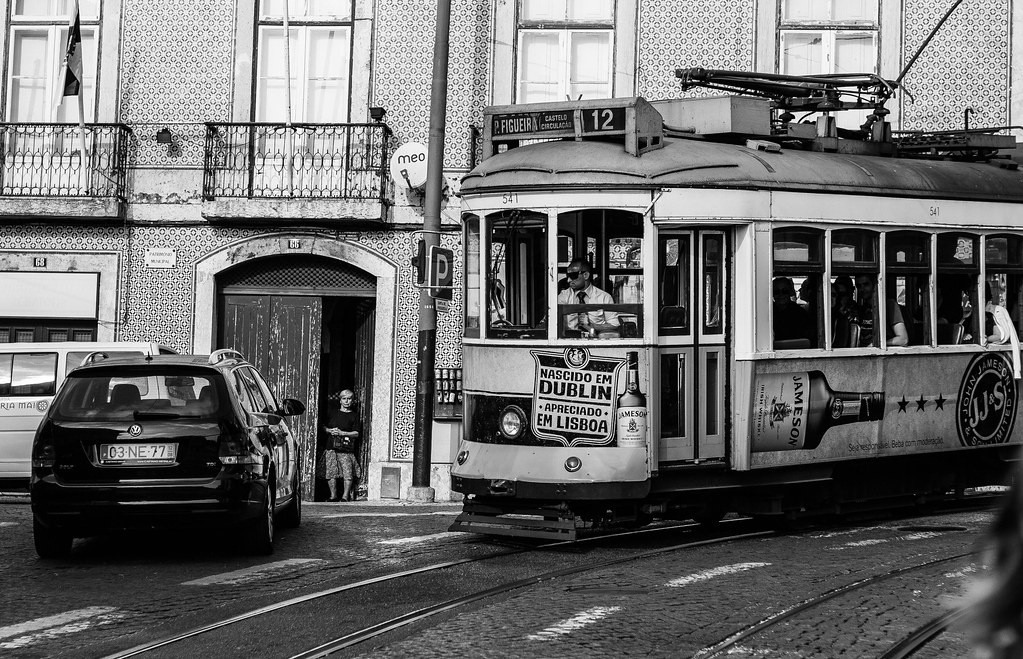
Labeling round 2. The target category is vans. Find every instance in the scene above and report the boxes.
[2,340,181,483]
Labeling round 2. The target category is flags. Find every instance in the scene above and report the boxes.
[58,0,82,107]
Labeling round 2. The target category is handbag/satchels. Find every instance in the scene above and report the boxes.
[333,435,355,452]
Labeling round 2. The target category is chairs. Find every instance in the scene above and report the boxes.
[90,384,211,407]
[659,306,1023,350]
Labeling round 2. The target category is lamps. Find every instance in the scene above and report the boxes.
[370,107,387,121]
[157,129,172,143]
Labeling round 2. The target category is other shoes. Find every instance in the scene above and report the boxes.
[326,497,348,503]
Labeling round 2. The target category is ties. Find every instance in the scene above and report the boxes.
[576,292,590,331]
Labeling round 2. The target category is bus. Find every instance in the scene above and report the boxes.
[445,0,1023,543]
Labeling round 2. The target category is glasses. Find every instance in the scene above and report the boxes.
[566,271,585,280]
[774,288,792,295]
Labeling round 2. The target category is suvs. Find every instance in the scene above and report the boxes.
[30,349,307,556]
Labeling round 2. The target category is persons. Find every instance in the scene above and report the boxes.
[535,258,621,338]
[773,234,1010,345]
[324,389,362,503]
